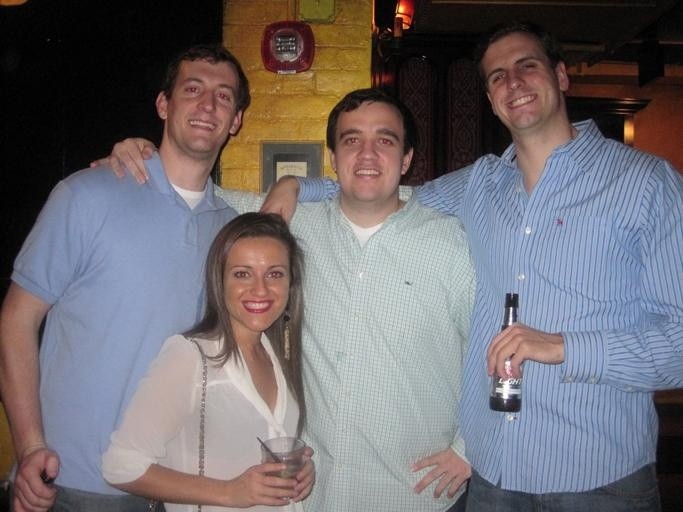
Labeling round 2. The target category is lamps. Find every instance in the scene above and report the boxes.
[372,0,417,72]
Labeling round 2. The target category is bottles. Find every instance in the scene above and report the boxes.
[488,293,524,412]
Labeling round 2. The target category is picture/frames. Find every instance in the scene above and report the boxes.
[261,142,324,193]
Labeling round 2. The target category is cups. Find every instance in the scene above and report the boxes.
[260,437,306,500]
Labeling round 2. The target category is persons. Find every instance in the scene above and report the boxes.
[99,211,318,512]
[88,87,474,512]
[0,39,253,512]
[257,20,683,512]
